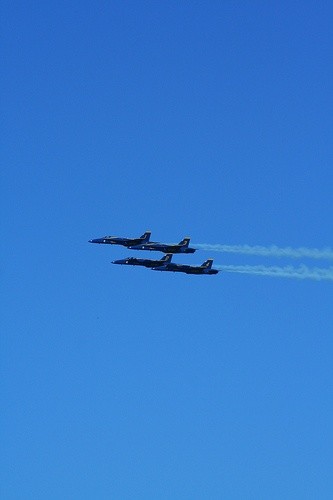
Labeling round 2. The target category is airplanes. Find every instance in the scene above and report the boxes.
[109,253,221,275]
[87,232,197,255]
